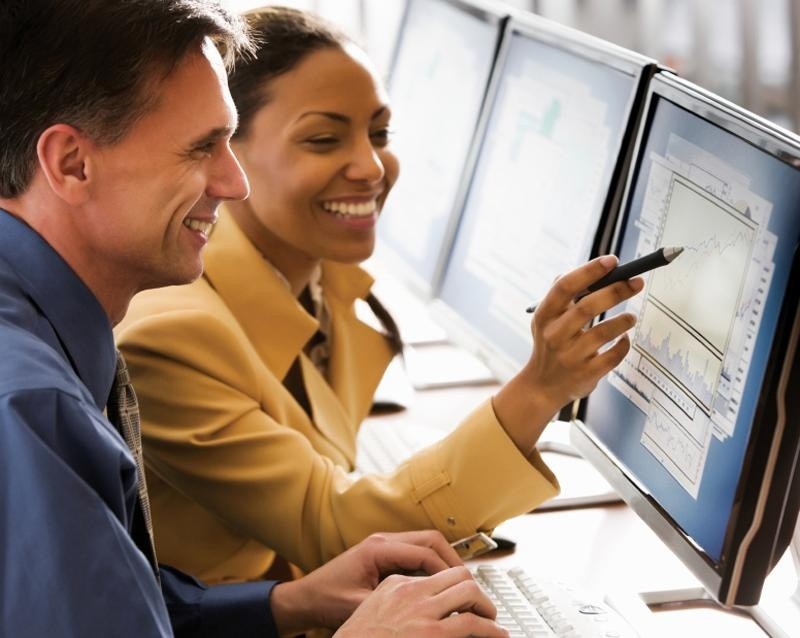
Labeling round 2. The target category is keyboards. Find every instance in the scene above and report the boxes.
[472,563,581,638]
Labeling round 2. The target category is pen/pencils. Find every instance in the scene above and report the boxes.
[526,246,685,313]
[368,402,407,417]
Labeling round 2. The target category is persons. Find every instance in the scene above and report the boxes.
[0,1,510,638]
[113,7,646,638]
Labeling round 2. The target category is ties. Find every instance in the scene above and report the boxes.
[107,349,160,589]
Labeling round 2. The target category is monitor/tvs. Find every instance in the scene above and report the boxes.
[424,8,657,422]
[374,0,514,304]
[563,71,799,609]
